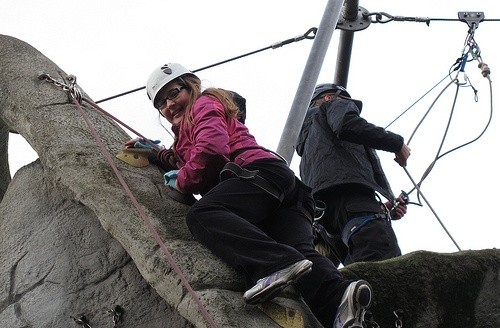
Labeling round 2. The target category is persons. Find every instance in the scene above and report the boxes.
[123,63,373,328]
[297,83,411,267]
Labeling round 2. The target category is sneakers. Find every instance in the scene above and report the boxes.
[333,279,373,328]
[243,260,313,304]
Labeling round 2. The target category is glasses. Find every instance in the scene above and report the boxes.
[155,86,184,109]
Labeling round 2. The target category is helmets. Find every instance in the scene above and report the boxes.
[145,62,193,108]
[312,82,351,99]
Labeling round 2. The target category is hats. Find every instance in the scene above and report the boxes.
[318,92,362,115]
